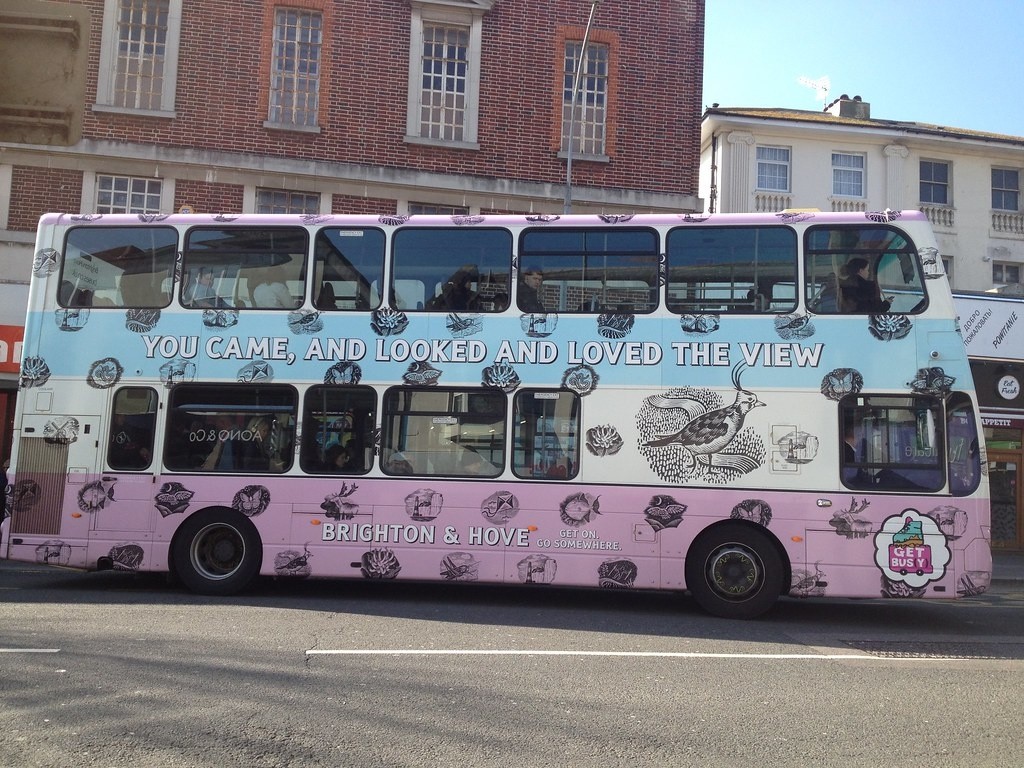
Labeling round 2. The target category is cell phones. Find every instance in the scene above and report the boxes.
[889,296,895,300]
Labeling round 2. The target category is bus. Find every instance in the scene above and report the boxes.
[1,208,993,621]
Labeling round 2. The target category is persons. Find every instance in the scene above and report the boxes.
[111,408,573,482]
[843,421,876,488]
[0,459,10,547]
[120,247,894,313]
